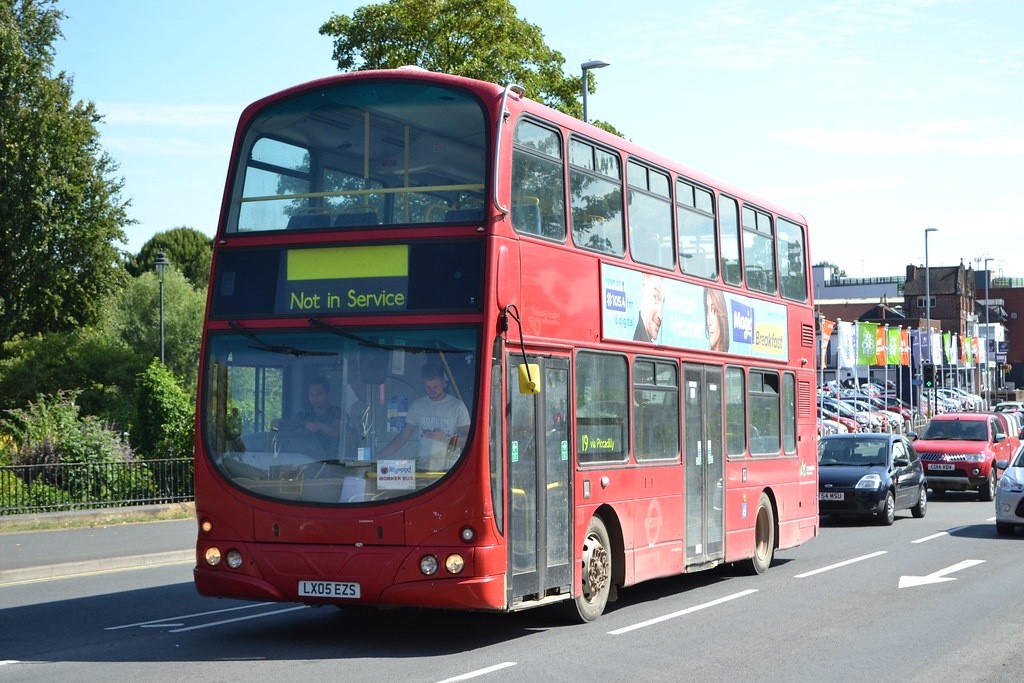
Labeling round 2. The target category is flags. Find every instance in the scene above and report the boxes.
[821,318,986,369]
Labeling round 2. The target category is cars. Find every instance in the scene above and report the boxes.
[819,432,929,526]
[816,377,1024,449]
[990,447,1024,537]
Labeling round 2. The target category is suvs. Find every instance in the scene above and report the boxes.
[909,410,1021,502]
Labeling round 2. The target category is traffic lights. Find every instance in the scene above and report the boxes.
[923,363,935,388]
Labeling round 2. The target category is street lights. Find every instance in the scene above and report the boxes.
[580,61,612,123]
[924,228,939,420]
[153,248,171,365]
[985,258,996,410]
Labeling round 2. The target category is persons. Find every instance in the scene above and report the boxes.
[934,421,987,439]
[703,286,729,352]
[632,272,667,343]
[271,361,474,472]
[828,441,853,462]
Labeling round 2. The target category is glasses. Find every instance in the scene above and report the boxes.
[649,292,666,304]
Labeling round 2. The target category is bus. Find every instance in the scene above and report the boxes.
[194,65,820,624]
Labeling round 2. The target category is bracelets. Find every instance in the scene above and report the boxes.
[444,434,452,442]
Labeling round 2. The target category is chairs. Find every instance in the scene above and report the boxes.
[333,206,378,227]
[423,197,785,298]
[286,207,330,229]
